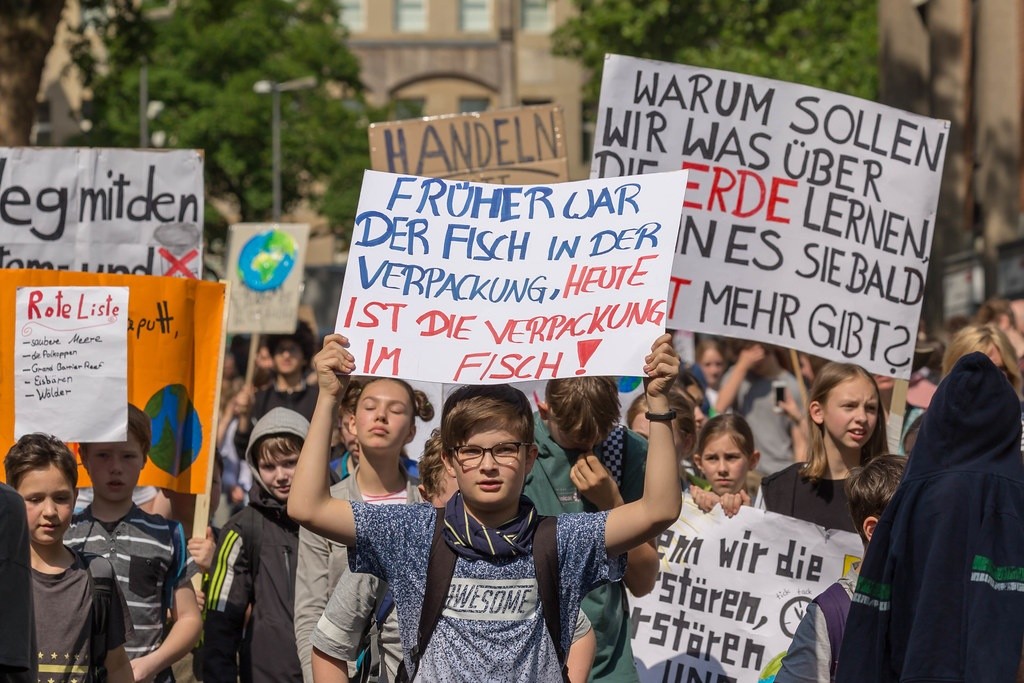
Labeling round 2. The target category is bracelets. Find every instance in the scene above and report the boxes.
[644,410,677,422]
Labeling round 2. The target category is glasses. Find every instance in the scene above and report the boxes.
[446,441,532,467]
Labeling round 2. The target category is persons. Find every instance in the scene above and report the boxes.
[833,351,1022,682]
[297,428,466,683]
[8,251,1022,561]
[523,375,661,683]
[771,453,914,683]
[283,335,684,683]
[292,375,438,683]
[2,432,138,683]
[64,402,209,682]
[204,405,310,682]
